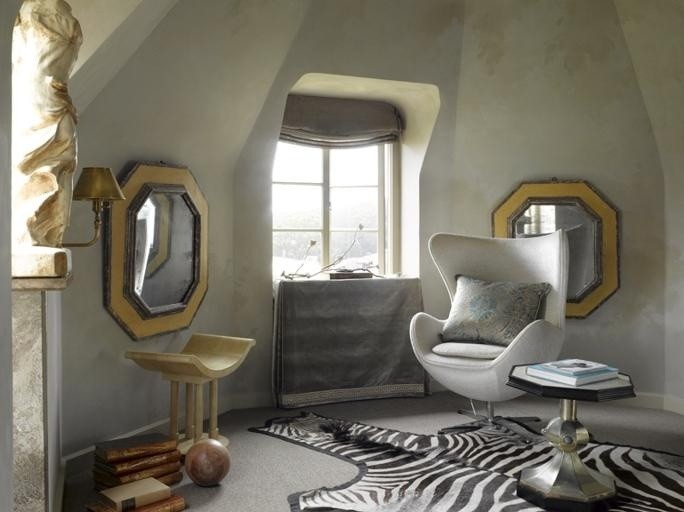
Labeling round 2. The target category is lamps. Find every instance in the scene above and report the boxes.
[61,164,126,248]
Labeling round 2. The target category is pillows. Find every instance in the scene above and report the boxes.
[436,272,552,348]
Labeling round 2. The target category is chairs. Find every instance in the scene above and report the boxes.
[407,227,572,442]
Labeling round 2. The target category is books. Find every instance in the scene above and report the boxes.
[526,358,619,387]
[84,433,187,512]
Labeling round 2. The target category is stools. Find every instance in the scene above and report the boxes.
[122,333,256,458]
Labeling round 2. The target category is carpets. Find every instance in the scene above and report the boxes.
[250,406,683,512]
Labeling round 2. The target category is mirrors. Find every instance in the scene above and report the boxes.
[100,160,210,343]
[491,177,623,320]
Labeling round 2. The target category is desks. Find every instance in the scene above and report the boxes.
[503,361,636,511]
